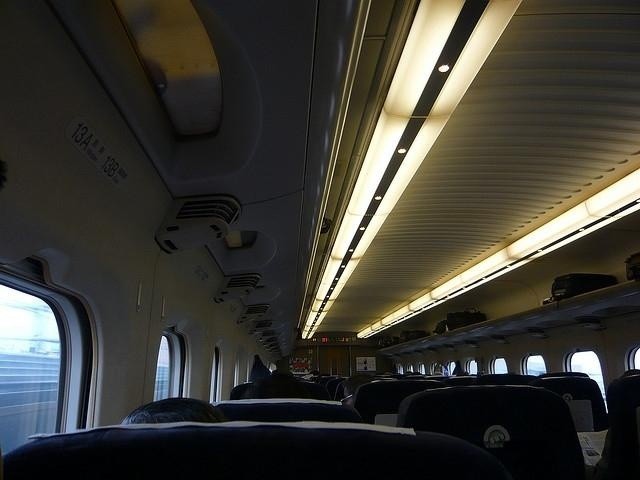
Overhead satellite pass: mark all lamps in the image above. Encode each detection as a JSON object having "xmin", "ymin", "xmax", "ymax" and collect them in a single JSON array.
[
  {"xmin": 297, "ymin": 0, "xmax": 523, "ymax": 342},
  {"xmin": 357, "ymin": 169, "xmax": 640, "ymax": 340}
]
